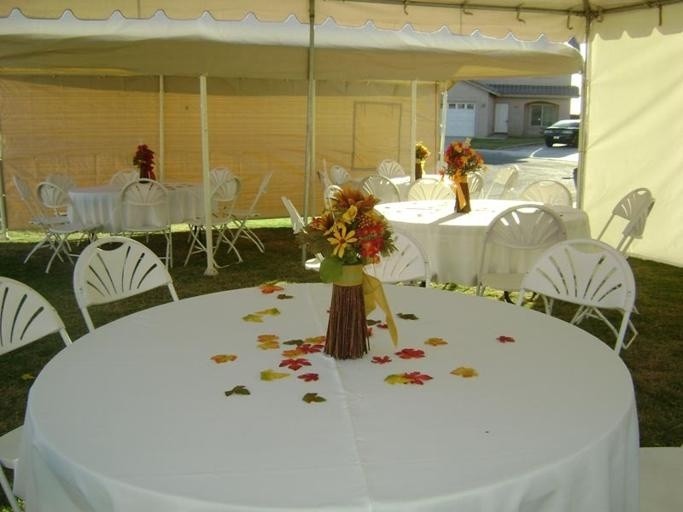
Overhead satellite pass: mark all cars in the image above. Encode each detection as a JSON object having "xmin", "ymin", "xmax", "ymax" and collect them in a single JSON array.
[{"xmin": 543, "ymin": 118, "xmax": 579, "ymax": 148}]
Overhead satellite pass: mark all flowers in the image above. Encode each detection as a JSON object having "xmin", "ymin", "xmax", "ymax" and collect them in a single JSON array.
[
  {"xmin": 133, "ymin": 144, "xmax": 154, "ymax": 164},
  {"xmin": 294, "ymin": 188, "xmax": 397, "ymax": 283},
  {"xmin": 415, "ymin": 143, "xmax": 430, "ymax": 162},
  {"xmin": 444, "ymin": 141, "xmax": 489, "ymax": 176}
]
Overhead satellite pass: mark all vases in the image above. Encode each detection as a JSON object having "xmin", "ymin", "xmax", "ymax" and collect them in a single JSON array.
[
  {"xmin": 139, "ymin": 165, "xmax": 149, "ymax": 183},
  {"xmin": 322, "ymin": 264, "xmax": 371, "ymax": 361}
]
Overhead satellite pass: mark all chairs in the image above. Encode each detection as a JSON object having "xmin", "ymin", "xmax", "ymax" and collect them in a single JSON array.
[
  {"xmin": 11, "ymin": 168, "xmax": 273, "ymax": 273},
  {"xmin": 1, "ymin": 277, "xmax": 72, "ymax": 512},
  {"xmin": 72, "ymin": 236, "xmax": 180, "ymax": 331}
]
